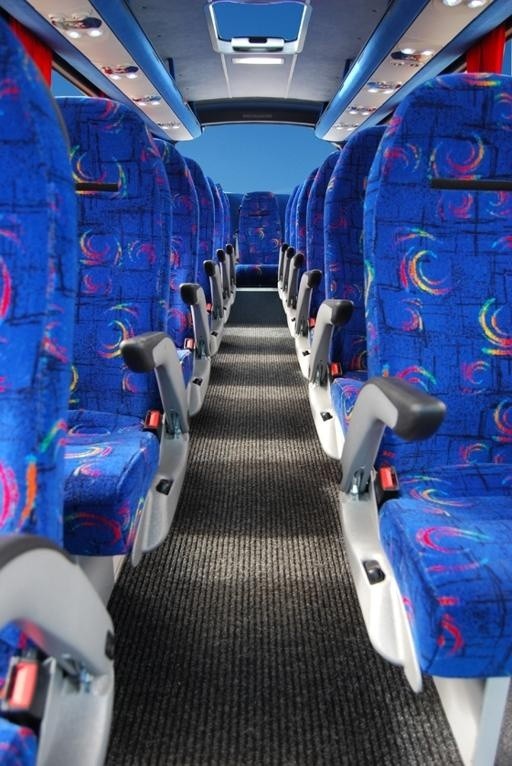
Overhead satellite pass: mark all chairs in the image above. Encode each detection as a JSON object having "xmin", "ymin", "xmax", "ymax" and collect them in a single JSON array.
[
  {"xmin": 234, "ymin": 192, "xmax": 281, "ymax": 285},
  {"xmin": 0, "ymin": 24, "xmax": 116, "ymax": 761},
  {"xmin": 336, "ymin": 71, "xmax": 512, "ymax": 766},
  {"xmin": 279, "ymin": 125, "xmax": 384, "ymax": 460},
  {"xmin": 153, "ymin": 140, "xmax": 233, "ymax": 419},
  {"xmin": 55, "ymin": 96, "xmax": 188, "ymax": 601}
]
